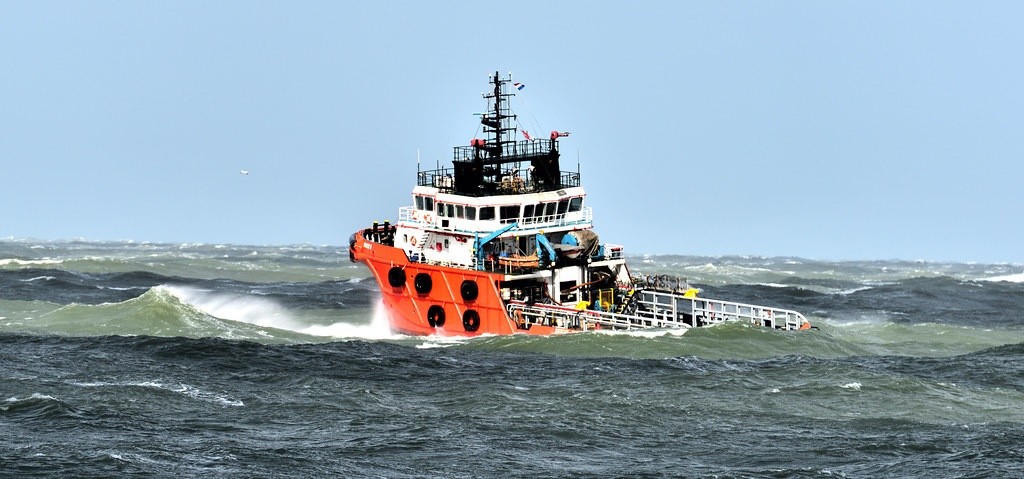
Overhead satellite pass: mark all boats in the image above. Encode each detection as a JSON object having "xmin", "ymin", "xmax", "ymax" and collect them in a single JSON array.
[{"xmin": 349, "ymin": 68, "xmax": 809, "ymax": 348}]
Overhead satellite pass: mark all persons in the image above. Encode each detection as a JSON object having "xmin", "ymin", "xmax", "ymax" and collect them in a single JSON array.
[{"xmin": 585, "ymin": 295, "xmax": 603, "ymax": 330}]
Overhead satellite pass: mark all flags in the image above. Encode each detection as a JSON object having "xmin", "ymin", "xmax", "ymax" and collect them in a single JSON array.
[{"xmin": 513, "ymin": 82, "xmax": 525, "ymax": 91}]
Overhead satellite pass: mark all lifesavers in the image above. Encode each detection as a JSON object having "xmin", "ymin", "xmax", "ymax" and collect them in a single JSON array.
[
  {"xmin": 410, "ymin": 236, "xmax": 417, "ymax": 246},
  {"xmin": 424, "ymin": 214, "xmax": 432, "ymax": 223}
]
